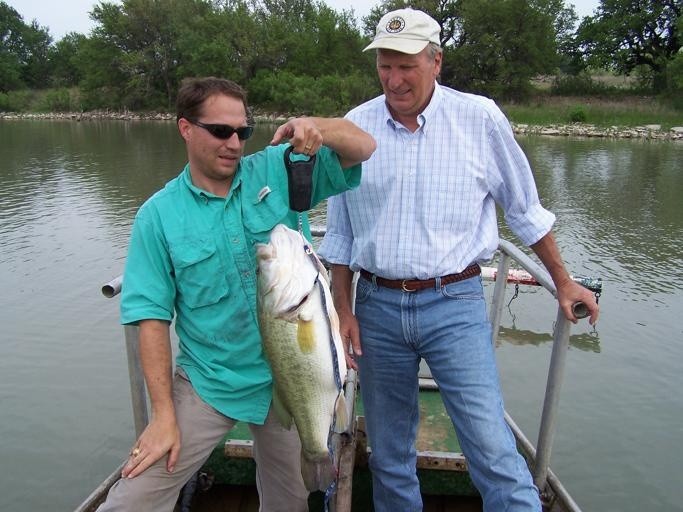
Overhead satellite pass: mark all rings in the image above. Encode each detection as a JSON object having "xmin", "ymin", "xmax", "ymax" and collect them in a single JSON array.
[
  {"xmin": 305, "ymin": 145, "xmax": 313, "ymax": 150},
  {"xmin": 133, "ymin": 448, "xmax": 141, "ymax": 456}
]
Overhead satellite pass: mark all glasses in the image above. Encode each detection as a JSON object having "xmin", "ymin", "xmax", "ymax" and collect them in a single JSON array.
[{"xmin": 195, "ymin": 117, "xmax": 253, "ymax": 140}]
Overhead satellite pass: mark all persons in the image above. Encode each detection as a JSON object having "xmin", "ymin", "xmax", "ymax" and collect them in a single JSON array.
[
  {"xmin": 318, "ymin": 8, "xmax": 599, "ymax": 512},
  {"xmin": 96, "ymin": 76, "xmax": 378, "ymax": 512}
]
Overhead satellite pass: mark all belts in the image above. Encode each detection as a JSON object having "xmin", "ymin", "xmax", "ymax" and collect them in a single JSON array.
[{"xmin": 359, "ymin": 262, "xmax": 482, "ymax": 292}]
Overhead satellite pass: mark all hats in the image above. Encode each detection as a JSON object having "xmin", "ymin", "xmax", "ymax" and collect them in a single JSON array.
[{"xmin": 362, "ymin": 7, "xmax": 442, "ymax": 56}]
